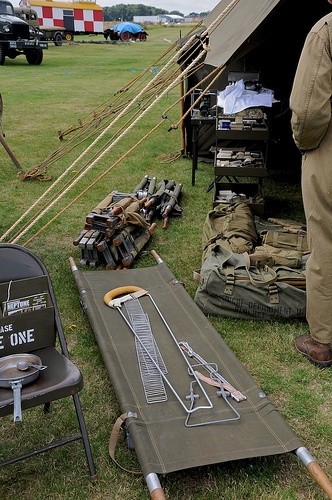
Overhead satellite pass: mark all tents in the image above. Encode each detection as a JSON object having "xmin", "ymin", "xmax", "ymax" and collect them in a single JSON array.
[{"xmin": 177, "ymin": 1, "xmax": 332, "ymax": 159}]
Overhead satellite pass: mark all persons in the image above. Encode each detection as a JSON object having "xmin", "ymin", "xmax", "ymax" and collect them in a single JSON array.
[{"xmin": 289, "ymin": 0, "xmax": 332, "ymax": 367}]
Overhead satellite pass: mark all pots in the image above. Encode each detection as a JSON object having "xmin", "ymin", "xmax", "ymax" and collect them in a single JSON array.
[{"xmin": 0, "ymin": 353, "xmax": 42, "ymax": 423}]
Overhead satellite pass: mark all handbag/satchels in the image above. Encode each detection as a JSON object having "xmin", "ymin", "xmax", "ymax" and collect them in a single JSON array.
[{"xmin": 194, "ymin": 202, "xmax": 307, "ymax": 320}]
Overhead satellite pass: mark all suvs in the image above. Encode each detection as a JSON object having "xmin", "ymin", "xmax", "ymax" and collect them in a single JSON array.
[{"xmin": 0, "ymin": 0, "xmax": 67, "ymax": 66}]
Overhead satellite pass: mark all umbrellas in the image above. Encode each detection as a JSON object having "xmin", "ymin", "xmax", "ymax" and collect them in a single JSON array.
[{"xmin": 110, "ymin": 22, "xmax": 145, "ymax": 44}]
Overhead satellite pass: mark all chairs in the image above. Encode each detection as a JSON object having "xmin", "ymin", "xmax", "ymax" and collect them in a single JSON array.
[{"xmin": 0, "ymin": 242, "xmax": 98, "ymax": 486}]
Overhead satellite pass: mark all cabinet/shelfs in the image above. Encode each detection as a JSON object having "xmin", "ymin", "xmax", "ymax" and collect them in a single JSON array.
[{"xmin": 214, "ymin": 106, "xmax": 271, "ymax": 218}]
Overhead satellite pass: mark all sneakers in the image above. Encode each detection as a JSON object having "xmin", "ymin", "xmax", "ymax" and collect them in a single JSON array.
[{"xmin": 295, "ymin": 333, "xmax": 332, "ymax": 367}]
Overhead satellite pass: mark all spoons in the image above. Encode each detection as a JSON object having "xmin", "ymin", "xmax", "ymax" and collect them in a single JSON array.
[{"xmin": 17, "ymin": 361, "xmax": 48, "ymax": 371}]
